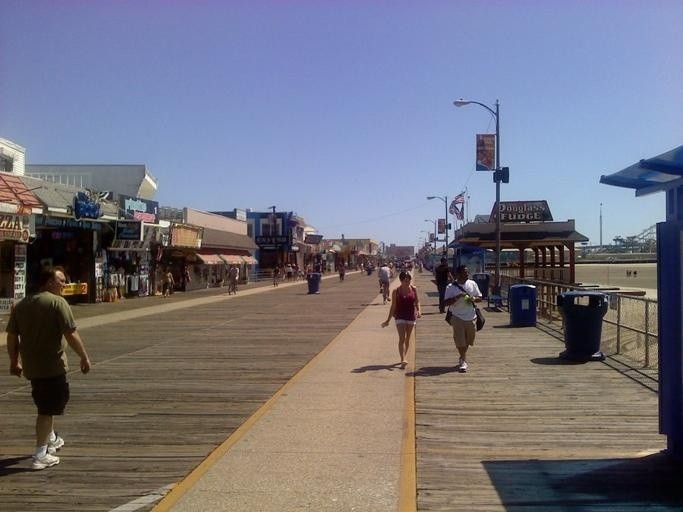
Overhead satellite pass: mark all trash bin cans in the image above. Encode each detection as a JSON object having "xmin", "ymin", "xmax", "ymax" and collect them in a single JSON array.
[
  {"xmin": 472, "ymin": 273, "xmax": 490, "ymax": 300},
  {"xmin": 557, "ymin": 291, "xmax": 608, "ymax": 361},
  {"xmin": 306, "ymin": 273, "xmax": 321, "ymax": 294},
  {"xmin": 509, "ymin": 284, "xmax": 537, "ymax": 326}
]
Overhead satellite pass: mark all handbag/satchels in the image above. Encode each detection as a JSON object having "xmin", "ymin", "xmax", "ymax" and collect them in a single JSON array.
[{"xmin": 451, "ymin": 281, "xmax": 486, "ymax": 333}]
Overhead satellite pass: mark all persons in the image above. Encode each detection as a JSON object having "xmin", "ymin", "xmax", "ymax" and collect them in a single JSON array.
[
  {"xmin": 227, "ymin": 264, "xmax": 239, "ymax": 291},
  {"xmin": 5, "ymin": 265, "xmax": 91, "ymax": 471},
  {"xmin": 160, "ymin": 268, "xmax": 175, "ymax": 296},
  {"xmin": 274, "ymin": 254, "xmax": 483, "ymax": 373}
]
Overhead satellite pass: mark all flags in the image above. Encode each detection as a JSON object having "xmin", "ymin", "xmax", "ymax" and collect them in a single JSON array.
[{"xmin": 449, "ymin": 193, "xmax": 465, "ymax": 220}]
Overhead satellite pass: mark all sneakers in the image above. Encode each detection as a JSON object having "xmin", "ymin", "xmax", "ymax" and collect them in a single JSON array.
[
  {"xmin": 29, "ymin": 452, "xmax": 61, "ymax": 470},
  {"xmin": 45, "ymin": 432, "xmax": 67, "ymax": 451},
  {"xmin": 459, "ymin": 356, "xmax": 470, "ymax": 371}
]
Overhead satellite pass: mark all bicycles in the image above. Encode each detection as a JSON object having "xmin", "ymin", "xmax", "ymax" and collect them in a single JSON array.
[
  {"xmin": 379, "ymin": 281, "xmax": 389, "ymax": 305},
  {"xmin": 273, "ymin": 275, "xmax": 279, "ymax": 287},
  {"xmin": 226, "ymin": 277, "xmax": 239, "ymax": 295}
]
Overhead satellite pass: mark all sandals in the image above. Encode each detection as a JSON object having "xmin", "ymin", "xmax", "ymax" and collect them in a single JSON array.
[{"xmin": 399, "ymin": 360, "xmax": 410, "ymax": 370}]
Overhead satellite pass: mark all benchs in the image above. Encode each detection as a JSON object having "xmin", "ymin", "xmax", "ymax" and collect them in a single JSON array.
[{"xmin": 487, "ymin": 294, "xmax": 510, "ymax": 313}]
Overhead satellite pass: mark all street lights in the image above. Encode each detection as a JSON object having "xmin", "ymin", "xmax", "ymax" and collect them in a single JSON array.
[
  {"xmin": 453, "ymin": 95, "xmax": 505, "ymax": 313},
  {"xmin": 267, "ymin": 206, "xmax": 279, "ymax": 267},
  {"xmin": 598, "ymin": 203, "xmax": 604, "ymax": 249},
  {"xmin": 424, "ymin": 218, "xmax": 438, "ymax": 257},
  {"xmin": 418, "ymin": 228, "xmax": 431, "ymax": 253},
  {"xmin": 427, "ymin": 194, "xmax": 449, "ymax": 266}
]
